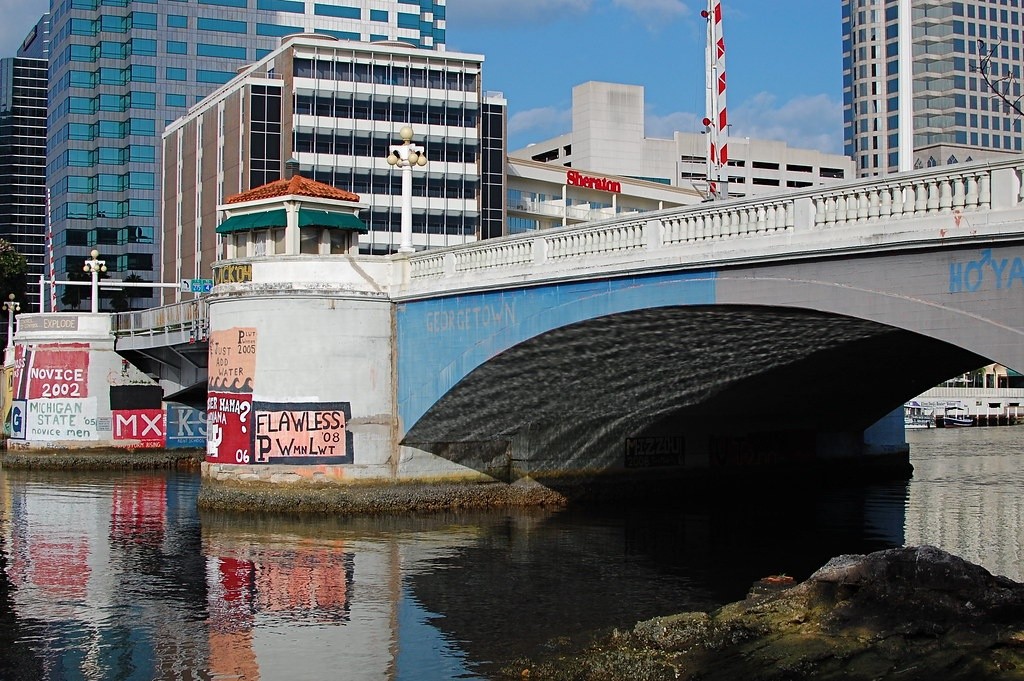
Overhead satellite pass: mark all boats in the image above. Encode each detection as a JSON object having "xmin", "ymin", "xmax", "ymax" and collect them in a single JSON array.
[
  {"xmin": 943, "ymin": 406, "xmax": 974, "ymax": 427},
  {"xmin": 904, "ymin": 401, "xmax": 937, "ymax": 429}
]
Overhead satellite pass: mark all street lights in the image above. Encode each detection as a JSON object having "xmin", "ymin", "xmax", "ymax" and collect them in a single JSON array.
[
  {"xmin": 2, "ymin": 293, "xmax": 20, "ymax": 366},
  {"xmin": 388, "ymin": 126, "xmax": 426, "ymax": 250},
  {"xmin": 83, "ymin": 250, "xmax": 107, "ymax": 311}
]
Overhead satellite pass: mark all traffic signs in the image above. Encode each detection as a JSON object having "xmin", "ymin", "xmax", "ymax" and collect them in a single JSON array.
[{"xmin": 180, "ymin": 279, "xmax": 190, "ymax": 292}]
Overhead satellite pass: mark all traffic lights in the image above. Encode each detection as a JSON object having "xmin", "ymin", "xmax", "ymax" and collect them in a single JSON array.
[
  {"xmin": 199, "ymin": 318, "xmax": 206, "ymax": 329},
  {"xmin": 192, "ymin": 319, "xmax": 200, "ymax": 330}
]
